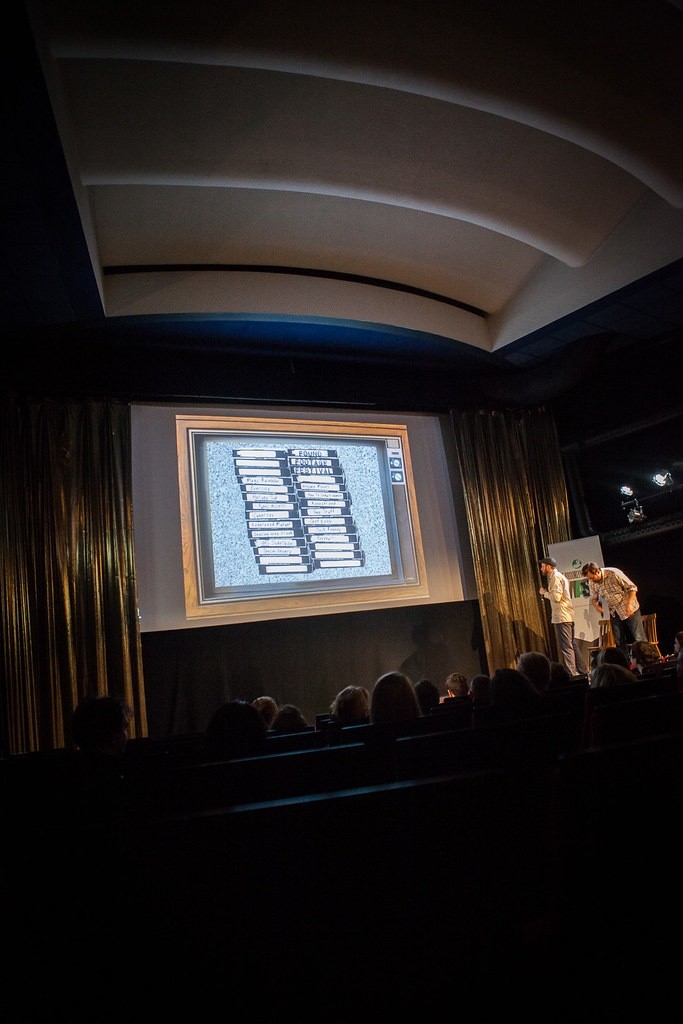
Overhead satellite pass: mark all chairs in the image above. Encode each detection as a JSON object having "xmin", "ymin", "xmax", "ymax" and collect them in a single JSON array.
[
  {"xmin": 588, "ymin": 619, "xmax": 617, "ymax": 673},
  {"xmin": 626, "ymin": 613, "xmax": 664, "ymax": 662}
]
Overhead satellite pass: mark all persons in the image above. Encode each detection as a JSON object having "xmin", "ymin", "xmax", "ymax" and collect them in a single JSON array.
[
  {"xmin": 467, "ymin": 674, "xmax": 490, "ymax": 708},
  {"xmin": 581, "ymin": 563, "xmax": 649, "ymax": 658},
  {"xmin": 413, "ymin": 680, "xmax": 440, "ymax": 710},
  {"xmin": 443, "ymin": 673, "xmax": 469, "ymax": 697},
  {"xmin": 674, "ymin": 630, "xmax": 683, "ymax": 662},
  {"xmin": 517, "ymin": 651, "xmax": 551, "ymax": 692},
  {"xmin": 630, "ymin": 640, "xmax": 660, "ymax": 675},
  {"xmin": 271, "ymin": 705, "xmax": 307, "ymax": 732},
  {"xmin": 549, "ymin": 662, "xmax": 570, "ymax": 683},
  {"xmin": 73, "ymin": 696, "xmax": 130, "ymax": 748},
  {"xmin": 250, "ymin": 696, "xmax": 277, "ymax": 731},
  {"xmin": 587, "ymin": 648, "xmax": 637, "ymax": 688},
  {"xmin": 328, "ymin": 685, "xmax": 370, "ymax": 722},
  {"xmin": 538, "ymin": 557, "xmax": 580, "ymax": 675},
  {"xmin": 204, "ymin": 700, "xmax": 268, "ymax": 759},
  {"xmin": 489, "ymin": 668, "xmax": 539, "ymax": 706},
  {"xmin": 370, "ymin": 672, "xmax": 422, "ymax": 726}
]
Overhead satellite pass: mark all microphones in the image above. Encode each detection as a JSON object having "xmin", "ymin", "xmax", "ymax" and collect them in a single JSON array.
[
  {"xmin": 540, "ymin": 585, "xmax": 546, "ymax": 602},
  {"xmin": 597, "ymin": 601, "xmax": 604, "ymax": 619}
]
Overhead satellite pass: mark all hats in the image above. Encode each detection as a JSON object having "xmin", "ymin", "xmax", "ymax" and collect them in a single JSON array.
[{"xmin": 538, "ymin": 557, "xmax": 556, "ymax": 566}]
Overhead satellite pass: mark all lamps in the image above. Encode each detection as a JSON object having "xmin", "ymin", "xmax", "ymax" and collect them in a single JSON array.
[
  {"xmin": 653, "ymin": 469, "xmax": 677, "ymax": 486},
  {"xmin": 627, "ymin": 509, "xmax": 648, "ymax": 524},
  {"xmin": 620, "ymin": 476, "xmax": 638, "ymax": 496}
]
